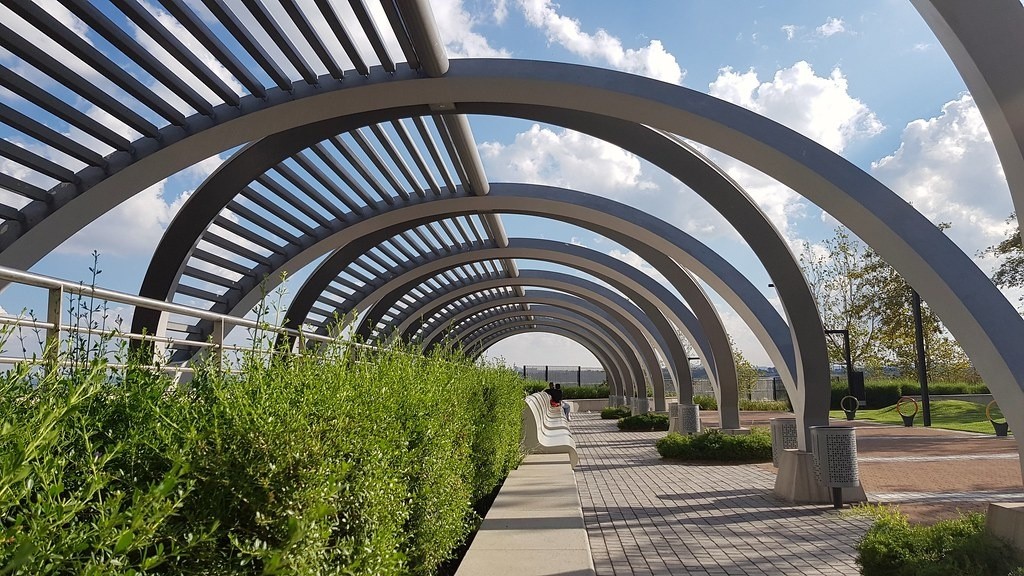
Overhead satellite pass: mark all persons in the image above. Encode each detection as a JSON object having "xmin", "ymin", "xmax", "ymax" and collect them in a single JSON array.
[{"xmin": 544, "ymin": 382, "xmax": 573, "ymax": 422}]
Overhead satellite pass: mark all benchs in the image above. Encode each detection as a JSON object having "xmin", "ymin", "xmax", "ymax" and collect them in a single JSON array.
[{"xmin": 524, "ymin": 391, "xmax": 579, "ymax": 469}]
